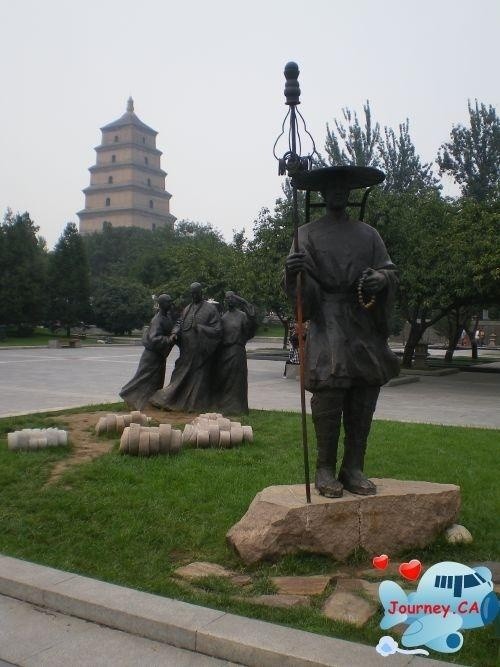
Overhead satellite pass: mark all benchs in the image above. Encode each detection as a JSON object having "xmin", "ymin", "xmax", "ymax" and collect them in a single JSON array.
[{"xmin": 47, "ymin": 338, "xmax": 82, "ymax": 348}]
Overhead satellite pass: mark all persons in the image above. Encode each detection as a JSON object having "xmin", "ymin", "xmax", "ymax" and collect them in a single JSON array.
[
  {"xmin": 213, "ymin": 291, "xmax": 257, "ymax": 416},
  {"xmin": 283, "ymin": 323, "xmax": 300, "ymax": 377},
  {"xmin": 119, "ymin": 294, "xmax": 174, "ymax": 411},
  {"xmin": 281, "ymin": 166, "xmax": 400, "ymax": 495},
  {"xmin": 461, "ymin": 327, "xmax": 486, "ymax": 347},
  {"xmin": 150, "ymin": 282, "xmax": 224, "ymax": 412}
]
